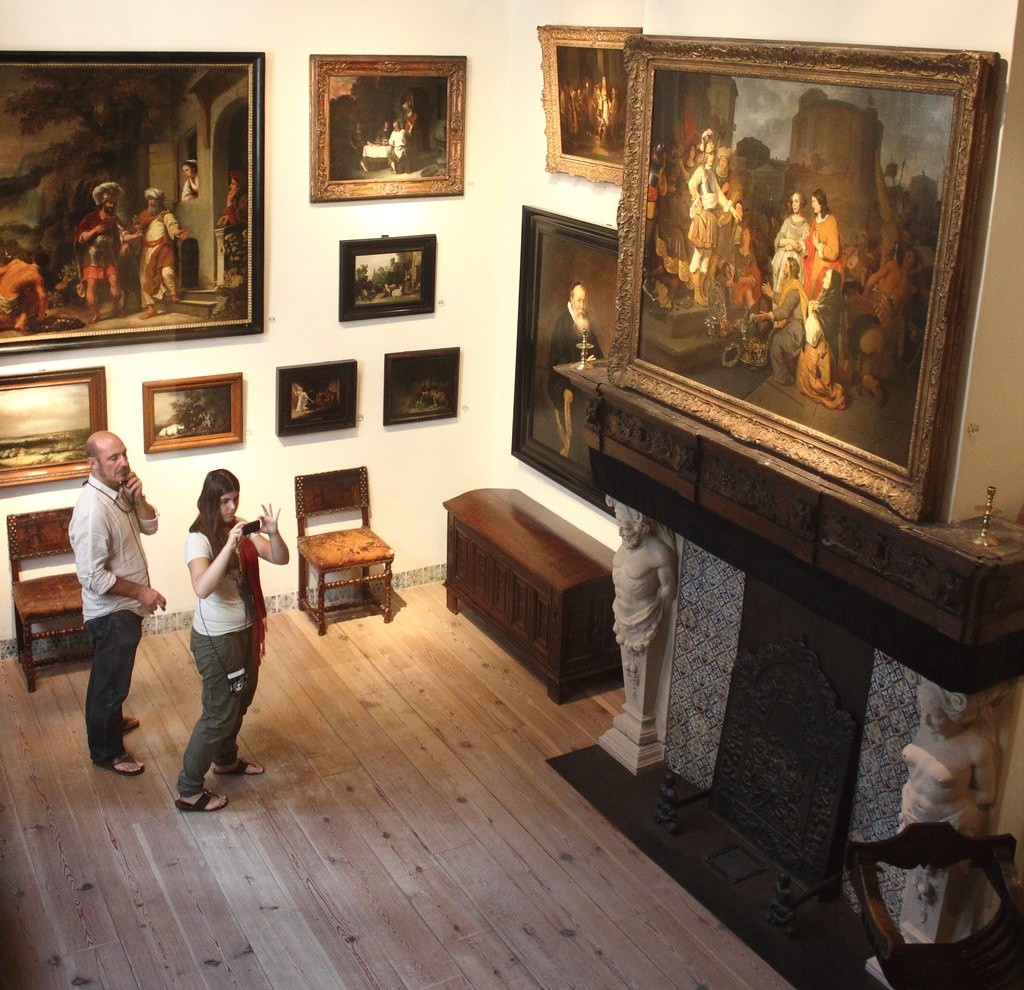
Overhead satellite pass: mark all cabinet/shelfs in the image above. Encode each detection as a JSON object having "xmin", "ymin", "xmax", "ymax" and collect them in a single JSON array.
[{"xmin": 442, "ymin": 488, "xmax": 624, "ymax": 706}]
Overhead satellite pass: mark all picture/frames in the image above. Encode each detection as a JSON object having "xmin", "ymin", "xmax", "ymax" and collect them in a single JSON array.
[
  {"xmin": 383, "ymin": 347, "xmax": 460, "ymax": 426},
  {"xmin": 510, "ymin": 204, "xmax": 617, "ymax": 520},
  {"xmin": 142, "ymin": 372, "xmax": 243, "ymax": 454},
  {"xmin": 0, "ymin": 365, "xmax": 109, "ymax": 487},
  {"xmin": 607, "ymin": 33, "xmax": 1000, "ymax": 526},
  {"xmin": 537, "ymin": 24, "xmax": 644, "ymax": 186},
  {"xmin": 0, "ymin": 51, "xmax": 266, "ymax": 357},
  {"xmin": 275, "ymin": 358, "xmax": 357, "ymax": 437},
  {"xmin": 309, "ymin": 53, "xmax": 468, "ymax": 204},
  {"xmin": 338, "ymin": 233, "xmax": 437, "ymax": 324}
]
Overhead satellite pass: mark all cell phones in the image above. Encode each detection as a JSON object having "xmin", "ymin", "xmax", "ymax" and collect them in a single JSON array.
[{"xmin": 242, "ymin": 521, "xmax": 260, "ymax": 535}]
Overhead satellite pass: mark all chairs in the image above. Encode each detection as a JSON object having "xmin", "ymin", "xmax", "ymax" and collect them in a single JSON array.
[
  {"xmin": 6, "ymin": 507, "xmax": 95, "ymax": 694},
  {"xmin": 294, "ymin": 465, "xmax": 395, "ymax": 637}
]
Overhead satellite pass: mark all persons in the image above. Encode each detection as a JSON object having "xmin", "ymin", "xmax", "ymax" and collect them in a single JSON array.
[
  {"xmin": 612, "ymin": 504, "xmax": 676, "ymax": 652},
  {"xmin": 902, "ymin": 682, "xmax": 996, "ymax": 829},
  {"xmin": 176, "ymin": 469, "xmax": 289, "ymax": 810},
  {"xmin": 68, "ymin": 431, "xmax": 166, "ymax": 776}
]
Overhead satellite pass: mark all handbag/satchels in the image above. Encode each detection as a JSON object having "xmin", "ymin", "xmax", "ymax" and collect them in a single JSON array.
[{"xmin": 225, "ymin": 668, "xmax": 250, "ymax": 697}]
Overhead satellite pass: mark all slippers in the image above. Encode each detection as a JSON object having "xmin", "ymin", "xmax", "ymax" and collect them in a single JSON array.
[
  {"xmin": 121, "ymin": 716, "xmax": 140, "ymax": 730},
  {"xmin": 213, "ymin": 758, "xmax": 265, "ymax": 776},
  {"xmin": 92, "ymin": 753, "xmax": 145, "ymax": 776},
  {"xmin": 175, "ymin": 788, "xmax": 228, "ymax": 811}
]
